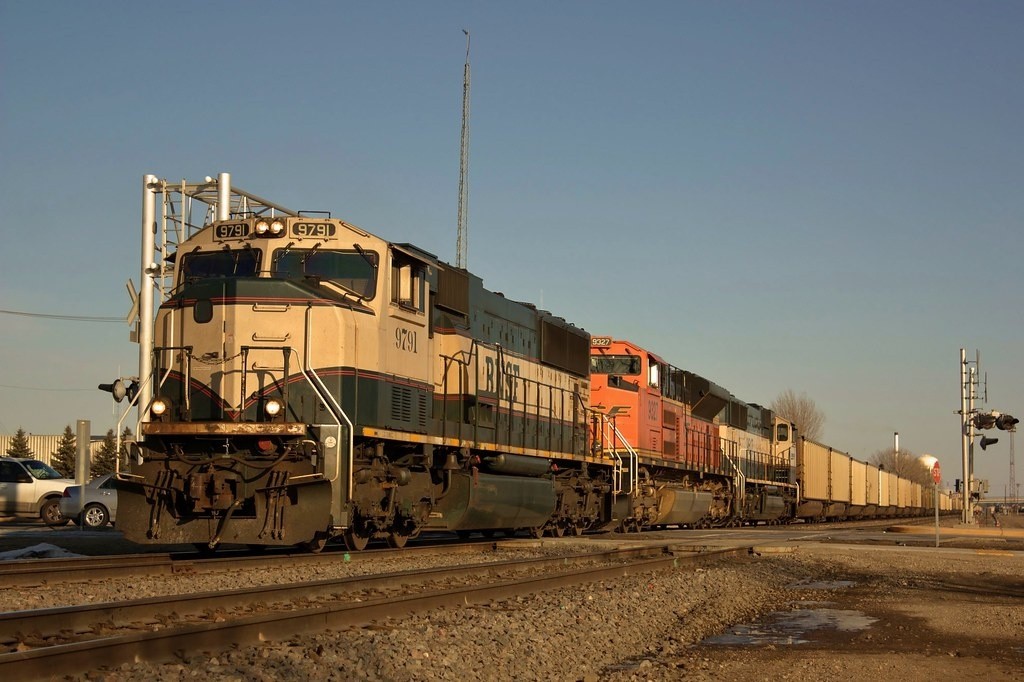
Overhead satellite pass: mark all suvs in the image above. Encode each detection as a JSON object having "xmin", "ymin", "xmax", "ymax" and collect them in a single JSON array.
[{"xmin": 0, "ymin": 455, "xmax": 75, "ymax": 527}]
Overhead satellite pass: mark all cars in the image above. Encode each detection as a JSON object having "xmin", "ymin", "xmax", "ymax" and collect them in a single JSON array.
[{"xmin": 59, "ymin": 470, "xmax": 129, "ymax": 531}]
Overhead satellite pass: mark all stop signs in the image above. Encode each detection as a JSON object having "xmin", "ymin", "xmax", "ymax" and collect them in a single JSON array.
[{"xmin": 932, "ymin": 462, "xmax": 941, "ymax": 484}]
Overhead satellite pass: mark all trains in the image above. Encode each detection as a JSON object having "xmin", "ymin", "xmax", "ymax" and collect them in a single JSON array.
[{"xmin": 99, "ymin": 173, "xmax": 963, "ymax": 559}]
